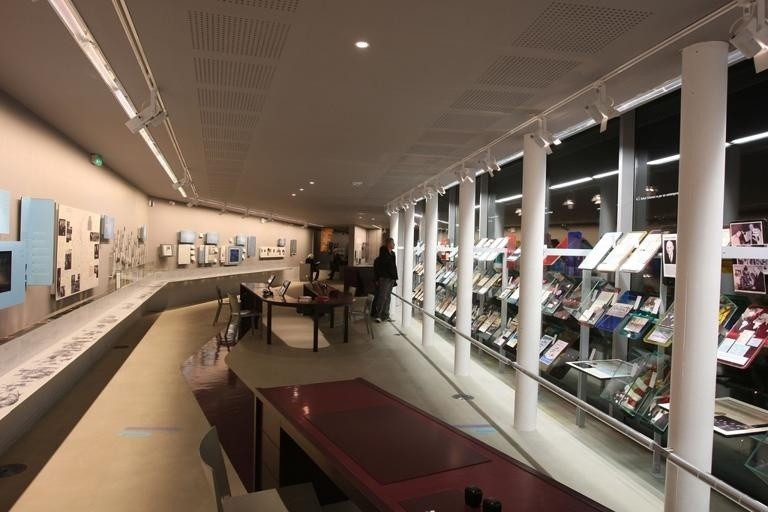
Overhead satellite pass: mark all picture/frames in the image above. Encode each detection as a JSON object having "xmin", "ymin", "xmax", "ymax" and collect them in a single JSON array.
[
  {"xmin": 565, "ymin": 359, "xmax": 636, "ymax": 380},
  {"xmin": 268, "ymin": 273, "xmax": 276, "ymax": 288},
  {"xmin": 278, "ymin": 280, "xmax": 291, "ymax": 297},
  {"xmin": 657, "ymin": 397, "xmax": 768, "ymax": 437}
]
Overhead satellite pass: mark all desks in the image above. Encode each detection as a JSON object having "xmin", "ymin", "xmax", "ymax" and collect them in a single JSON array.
[
  {"xmin": 255, "ymin": 377, "xmax": 614, "ymax": 512},
  {"xmin": 240, "ymin": 282, "xmax": 356, "ymax": 353}
]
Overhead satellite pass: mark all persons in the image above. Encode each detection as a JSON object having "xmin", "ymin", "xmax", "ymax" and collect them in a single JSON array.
[
  {"xmin": 664, "ymin": 240, "xmax": 675, "ymax": 264},
  {"xmin": 731, "ymin": 230, "xmax": 741, "ymax": 244},
  {"xmin": 740, "ymin": 266, "xmax": 755, "ymax": 289},
  {"xmin": 370, "ymin": 245, "xmax": 387, "ymax": 318},
  {"xmin": 741, "ymin": 224, "xmax": 761, "ymax": 246},
  {"xmin": 375, "ymin": 238, "xmax": 398, "ymax": 322},
  {"xmin": 749, "ymin": 266, "xmax": 764, "ymax": 291}
]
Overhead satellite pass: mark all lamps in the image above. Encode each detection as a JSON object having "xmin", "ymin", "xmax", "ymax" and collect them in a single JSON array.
[
  {"xmin": 479, "ymin": 143, "xmax": 501, "ymax": 178},
  {"xmin": 728, "ymin": 0, "xmax": 768, "ymax": 73},
  {"xmin": 187, "ymin": 195, "xmax": 199, "ymax": 208},
  {"xmin": 91, "ymin": 153, "xmax": 103, "ymax": 166},
  {"xmin": 384, "ymin": 175, "xmax": 446, "ymax": 217},
  {"xmin": 584, "ymin": 78, "xmax": 619, "ymax": 132},
  {"xmin": 50, "ymin": 0, "xmax": 188, "ymax": 197},
  {"xmin": 454, "ymin": 158, "xmax": 474, "ymax": 184},
  {"xmin": 125, "ymin": 89, "xmax": 167, "ymax": 134},
  {"xmin": 172, "ymin": 170, "xmax": 192, "ymax": 190},
  {"xmin": 531, "ymin": 112, "xmax": 562, "ymax": 155}
]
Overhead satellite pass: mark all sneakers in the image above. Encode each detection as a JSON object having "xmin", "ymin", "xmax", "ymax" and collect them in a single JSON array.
[
  {"xmin": 382, "ymin": 316, "xmax": 396, "ymax": 322},
  {"xmin": 375, "ymin": 317, "xmax": 381, "ymax": 323}
]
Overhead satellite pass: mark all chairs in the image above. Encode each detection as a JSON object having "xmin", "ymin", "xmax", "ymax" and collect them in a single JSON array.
[
  {"xmin": 225, "ymin": 295, "xmax": 263, "ymax": 336},
  {"xmin": 199, "ymin": 425, "xmax": 322, "ymax": 512},
  {"xmin": 348, "ymin": 293, "xmax": 374, "ymax": 340},
  {"xmin": 213, "ymin": 286, "xmax": 242, "ymax": 326}
]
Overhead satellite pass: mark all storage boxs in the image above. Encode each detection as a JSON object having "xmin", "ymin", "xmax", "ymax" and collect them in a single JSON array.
[
  {"xmin": 599, "ymin": 346, "xmax": 672, "ymax": 433},
  {"xmin": 542, "ymin": 270, "xmax": 574, "ymax": 316},
  {"xmin": 564, "ymin": 278, "xmax": 614, "ymax": 325},
  {"xmin": 745, "ymin": 432, "xmax": 768, "ymax": 487}
]
